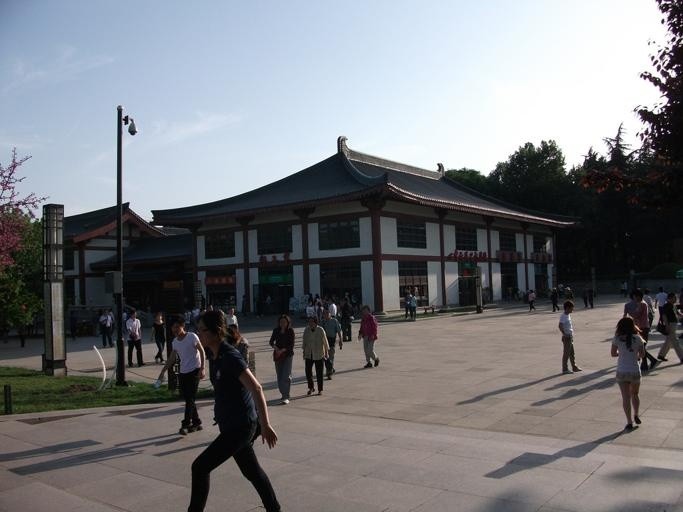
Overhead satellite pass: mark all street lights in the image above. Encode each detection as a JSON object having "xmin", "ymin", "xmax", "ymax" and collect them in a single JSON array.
[
  {"xmin": 113, "ymin": 104, "xmax": 140, "ymax": 385},
  {"xmin": 40, "ymin": 204, "xmax": 68, "ymax": 377}
]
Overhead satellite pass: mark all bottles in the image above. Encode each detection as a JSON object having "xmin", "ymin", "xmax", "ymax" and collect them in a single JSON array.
[{"xmin": 152, "ymin": 375, "xmax": 165, "ymax": 389}]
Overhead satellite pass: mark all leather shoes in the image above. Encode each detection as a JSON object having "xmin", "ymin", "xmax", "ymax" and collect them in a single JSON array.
[
  {"xmin": 641, "ymin": 366, "xmax": 648, "ymax": 371},
  {"xmin": 650, "ymin": 359, "xmax": 657, "ymax": 369},
  {"xmin": 658, "ymin": 355, "xmax": 667, "ymax": 361}
]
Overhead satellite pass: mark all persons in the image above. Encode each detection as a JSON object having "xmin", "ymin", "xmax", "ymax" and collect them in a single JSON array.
[
  {"xmin": 509, "ymin": 280, "xmax": 682, "ymax": 374},
  {"xmin": 611, "ymin": 318, "xmax": 646, "ymax": 430},
  {"xmin": 358, "ymin": 306, "xmax": 380, "ymax": 367},
  {"xmin": 405, "ymin": 293, "xmax": 416, "ymax": 321},
  {"xmin": 100, "ymin": 292, "xmax": 357, "ymax": 435},
  {"xmin": 187, "ymin": 309, "xmax": 281, "ymax": 512}
]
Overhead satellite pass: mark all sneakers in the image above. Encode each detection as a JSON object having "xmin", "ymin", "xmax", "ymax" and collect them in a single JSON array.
[
  {"xmin": 365, "ymin": 363, "xmax": 372, "ymax": 368},
  {"xmin": 573, "ymin": 367, "xmax": 581, "ymax": 372},
  {"xmin": 374, "ymin": 359, "xmax": 379, "ymax": 366},
  {"xmin": 189, "ymin": 425, "xmax": 202, "ymax": 431},
  {"xmin": 283, "ymin": 399, "xmax": 289, "ymax": 404},
  {"xmin": 180, "ymin": 425, "xmax": 189, "ymax": 435},
  {"xmin": 280, "ymin": 398, "xmax": 283, "ymax": 405},
  {"xmin": 318, "ymin": 391, "xmax": 322, "ymax": 395},
  {"xmin": 563, "ymin": 370, "xmax": 573, "ymax": 374},
  {"xmin": 308, "ymin": 389, "xmax": 315, "ymax": 395}
]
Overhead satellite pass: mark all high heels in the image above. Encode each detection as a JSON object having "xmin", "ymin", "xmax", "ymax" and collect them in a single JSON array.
[
  {"xmin": 625, "ymin": 424, "xmax": 633, "ymax": 431},
  {"xmin": 635, "ymin": 416, "xmax": 641, "ymax": 424}
]
